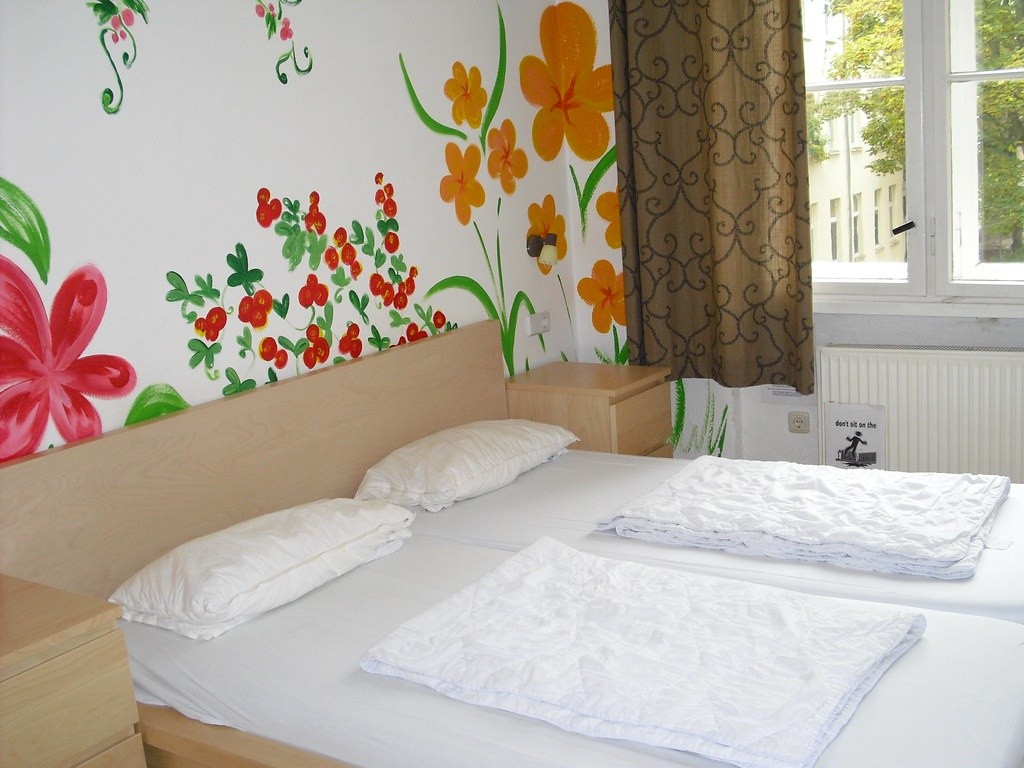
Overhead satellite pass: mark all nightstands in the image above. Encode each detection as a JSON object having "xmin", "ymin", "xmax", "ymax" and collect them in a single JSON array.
[
  {"xmin": 506, "ymin": 361, "xmax": 674, "ymax": 458},
  {"xmin": 0, "ymin": 573, "xmax": 147, "ymax": 768}
]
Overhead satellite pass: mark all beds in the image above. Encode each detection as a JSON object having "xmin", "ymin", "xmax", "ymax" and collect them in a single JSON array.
[{"xmin": 0, "ymin": 319, "xmax": 1024, "ymax": 768}]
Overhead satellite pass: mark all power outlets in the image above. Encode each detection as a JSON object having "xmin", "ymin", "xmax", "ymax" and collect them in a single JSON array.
[{"xmin": 788, "ymin": 411, "xmax": 810, "ymax": 434}]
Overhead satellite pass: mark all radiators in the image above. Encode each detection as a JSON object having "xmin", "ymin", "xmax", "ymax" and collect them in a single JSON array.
[{"xmin": 819, "ymin": 341, "xmax": 1024, "ymax": 483}]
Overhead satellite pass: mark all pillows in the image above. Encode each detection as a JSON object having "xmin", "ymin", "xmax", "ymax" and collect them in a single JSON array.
[
  {"xmin": 108, "ymin": 496, "xmax": 418, "ymax": 641},
  {"xmin": 354, "ymin": 416, "xmax": 580, "ymax": 514}
]
